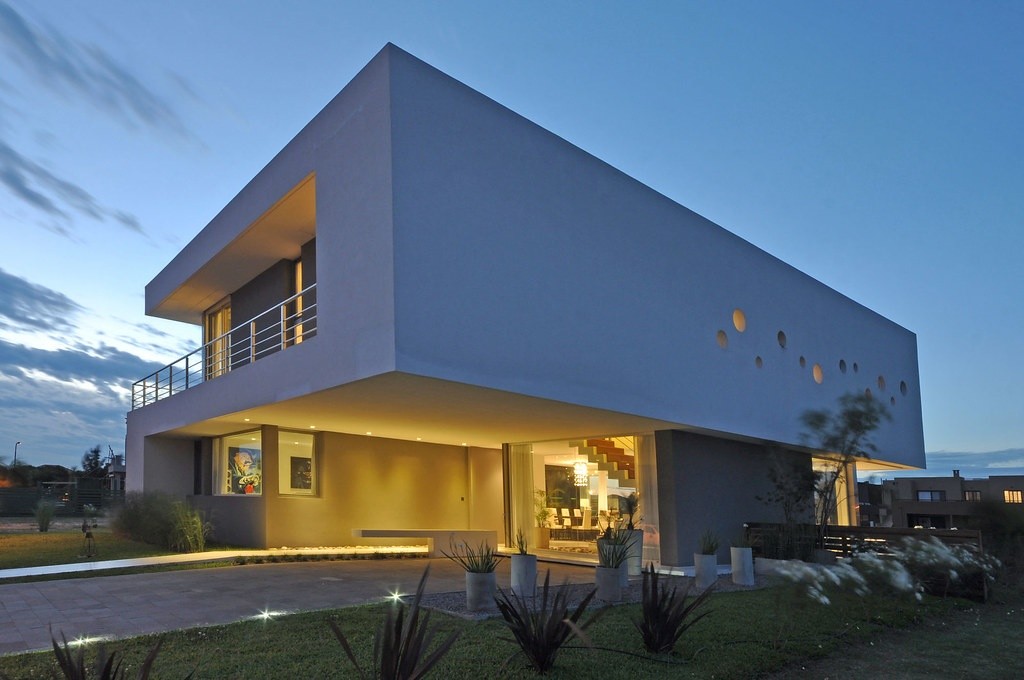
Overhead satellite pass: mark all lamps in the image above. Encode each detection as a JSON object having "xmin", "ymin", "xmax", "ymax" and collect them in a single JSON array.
[{"xmin": 572, "ymin": 462, "xmax": 588, "ymax": 487}]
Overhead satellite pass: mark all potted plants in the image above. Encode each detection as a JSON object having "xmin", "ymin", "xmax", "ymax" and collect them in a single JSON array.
[
  {"xmin": 594, "ymin": 532, "xmax": 640, "ymax": 600},
  {"xmin": 620, "ymin": 493, "xmax": 644, "ymax": 576},
  {"xmin": 440, "ymin": 532, "xmax": 504, "ymax": 610},
  {"xmin": 726, "ymin": 533, "xmax": 757, "ymax": 586},
  {"xmin": 533, "ymin": 487, "xmax": 568, "ymax": 548},
  {"xmin": 597, "ymin": 511, "xmax": 629, "ymax": 589},
  {"xmin": 692, "ymin": 529, "xmax": 720, "ymax": 589},
  {"xmin": 508, "ymin": 529, "xmax": 537, "ymax": 598}
]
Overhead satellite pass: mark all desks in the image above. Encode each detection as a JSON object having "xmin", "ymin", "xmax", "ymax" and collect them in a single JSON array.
[{"xmin": 554, "ymin": 515, "xmax": 599, "ymax": 541}]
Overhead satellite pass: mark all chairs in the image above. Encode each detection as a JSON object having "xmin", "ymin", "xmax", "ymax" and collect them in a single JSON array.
[
  {"xmin": 561, "ymin": 508, "xmax": 575, "ymax": 538},
  {"xmin": 573, "ymin": 508, "xmax": 583, "ymax": 538},
  {"xmin": 545, "ymin": 508, "xmax": 560, "ymax": 539},
  {"xmin": 545, "ymin": 514, "xmax": 567, "ymax": 540},
  {"xmin": 572, "ymin": 509, "xmax": 593, "ymax": 540}
]
